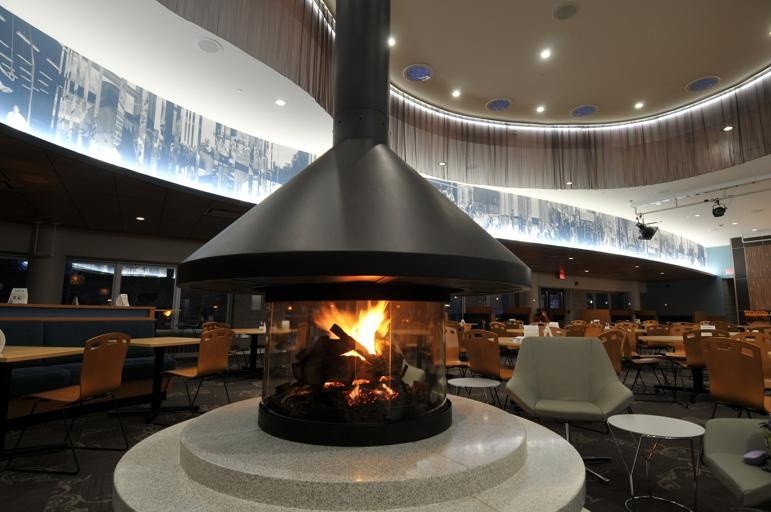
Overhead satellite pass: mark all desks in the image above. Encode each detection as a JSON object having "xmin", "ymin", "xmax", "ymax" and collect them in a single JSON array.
[
  {"xmin": 0, "ymin": 345, "xmax": 84, "ymax": 460},
  {"xmin": 393, "ymin": 329, "xmax": 431, "ymax": 368},
  {"xmin": 607, "ymin": 414, "xmax": 706, "ymax": 512},
  {"xmin": 107, "ymin": 336, "xmax": 210, "ymax": 424}
]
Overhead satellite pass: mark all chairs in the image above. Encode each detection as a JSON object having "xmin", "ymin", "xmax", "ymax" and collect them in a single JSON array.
[
  {"xmin": 5, "ymin": 333, "xmax": 131, "ymax": 474},
  {"xmin": 202, "ymin": 322, "xmax": 307, "ymax": 377},
  {"xmin": 446, "ymin": 320, "xmax": 771, "ymax": 506},
  {"xmin": 149, "ymin": 328, "xmax": 234, "ymax": 427}
]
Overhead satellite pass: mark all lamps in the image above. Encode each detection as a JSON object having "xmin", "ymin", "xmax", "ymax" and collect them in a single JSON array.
[
  {"xmin": 712, "ymin": 198, "xmax": 727, "ymax": 217},
  {"xmin": 636, "ymin": 213, "xmax": 658, "ymax": 240}
]
